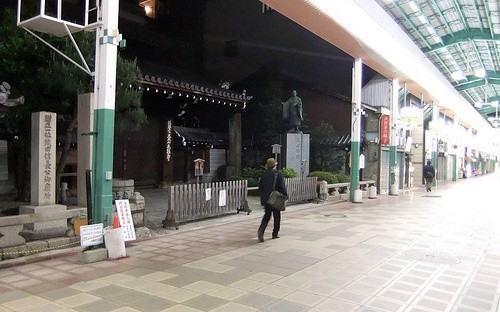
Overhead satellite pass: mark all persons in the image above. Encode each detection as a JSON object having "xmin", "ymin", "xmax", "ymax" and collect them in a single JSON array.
[
  {"xmin": 255, "ymin": 158, "xmax": 288, "ymax": 243},
  {"xmin": 280, "ymin": 88, "xmax": 304, "ymax": 133},
  {"xmin": 422, "ymin": 160, "xmax": 435, "ymax": 192}
]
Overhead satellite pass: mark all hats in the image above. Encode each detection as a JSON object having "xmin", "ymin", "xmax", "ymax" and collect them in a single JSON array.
[{"xmin": 264, "ymin": 158, "xmax": 278, "ymax": 169}]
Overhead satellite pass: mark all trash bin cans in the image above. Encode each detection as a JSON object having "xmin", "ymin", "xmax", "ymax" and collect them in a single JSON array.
[{"xmin": 112, "ymin": 178, "xmax": 134, "ymax": 205}]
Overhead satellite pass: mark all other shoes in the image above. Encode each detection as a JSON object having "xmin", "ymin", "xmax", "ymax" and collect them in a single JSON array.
[
  {"xmin": 258, "ymin": 228, "xmax": 264, "ymax": 241},
  {"xmin": 272, "ymin": 234, "xmax": 280, "ymax": 239}
]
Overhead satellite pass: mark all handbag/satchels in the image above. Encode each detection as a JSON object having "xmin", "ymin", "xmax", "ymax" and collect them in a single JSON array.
[
  {"xmin": 267, "ymin": 171, "xmax": 288, "ymax": 211},
  {"xmin": 425, "ymin": 172, "xmax": 434, "ymax": 178}
]
